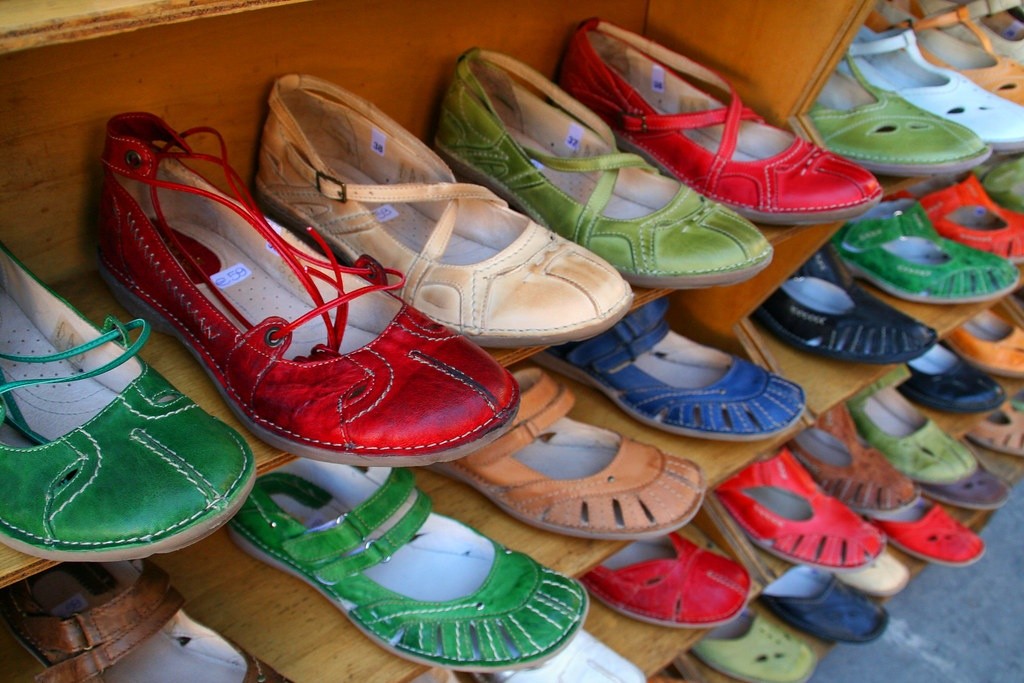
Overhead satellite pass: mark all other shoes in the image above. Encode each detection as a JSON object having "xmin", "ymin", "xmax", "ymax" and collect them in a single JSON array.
[
  {"xmin": 227, "ymin": 456, "xmax": 589, "ymax": 673},
  {"xmin": 427, "ymin": 367, "xmax": 707, "ymax": 541},
  {"xmin": 556, "ymin": 16, "xmax": 884, "ymax": 226},
  {"xmin": 691, "ymin": 2, "xmax": 1022, "ymax": 683},
  {"xmin": 1, "ymin": 244, "xmax": 257, "ymax": 561},
  {"xmin": 256, "ymin": 72, "xmax": 634, "ymax": 349},
  {"xmin": 435, "ymin": 48, "xmax": 773, "ymax": 289},
  {"xmin": 581, "ymin": 531, "xmax": 752, "ymax": 628},
  {"xmin": 473, "ymin": 628, "xmax": 646, "ymax": 683},
  {"xmin": 93, "ymin": 112, "xmax": 520, "ymax": 468},
  {"xmin": 0, "ymin": 561, "xmax": 294, "ymax": 683},
  {"xmin": 529, "ymin": 297, "xmax": 806, "ymax": 441}
]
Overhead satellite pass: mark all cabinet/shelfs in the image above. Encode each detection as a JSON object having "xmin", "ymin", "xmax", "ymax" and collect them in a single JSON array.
[{"xmin": 0, "ymin": 0, "xmax": 1024, "ymax": 681}]
